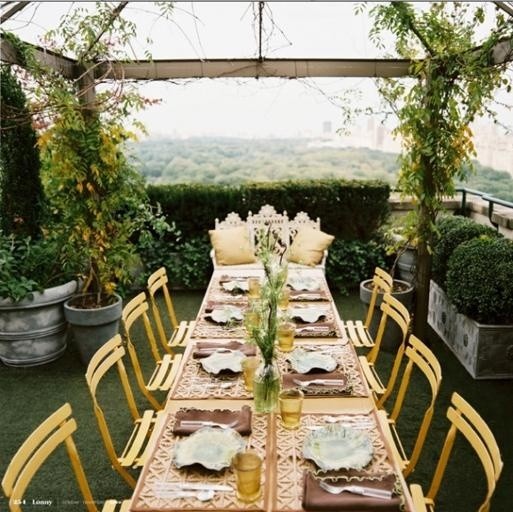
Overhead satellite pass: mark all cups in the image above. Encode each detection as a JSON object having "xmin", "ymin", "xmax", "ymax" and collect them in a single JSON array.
[
  {"xmin": 278, "ymin": 388, "xmax": 303, "ymax": 430},
  {"xmin": 232, "ymin": 452, "xmax": 266, "ymax": 501}
]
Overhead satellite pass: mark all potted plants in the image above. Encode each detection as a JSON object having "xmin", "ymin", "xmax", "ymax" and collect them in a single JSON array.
[
  {"xmin": 0, "ymin": 229, "xmax": 78, "ymax": 369},
  {"xmin": 40, "ymin": 76, "xmax": 154, "ymax": 362},
  {"xmin": 251, "ymin": 224, "xmax": 288, "ymax": 415}
]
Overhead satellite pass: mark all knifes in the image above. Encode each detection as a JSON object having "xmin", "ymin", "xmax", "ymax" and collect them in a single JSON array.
[{"xmin": 154, "ymin": 478, "xmax": 234, "ymax": 491}]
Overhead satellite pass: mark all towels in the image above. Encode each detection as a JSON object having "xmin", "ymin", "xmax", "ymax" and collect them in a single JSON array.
[
  {"xmin": 300, "ymin": 465, "xmax": 403, "ymax": 510},
  {"xmin": 203, "ymin": 299, "xmax": 247, "ymax": 316},
  {"xmin": 293, "ymin": 321, "xmax": 341, "ymax": 339},
  {"xmin": 170, "ymin": 403, "xmax": 252, "ymax": 435},
  {"xmin": 191, "ymin": 337, "xmax": 257, "ymax": 358},
  {"xmin": 287, "ymin": 288, "xmax": 330, "ymax": 302},
  {"xmin": 276, "ymin": 368, "xmax": 353, "ymax": 395},
  {"xmin": 217, "ymin": 273, "xmax": 261, "ymax": 285}
]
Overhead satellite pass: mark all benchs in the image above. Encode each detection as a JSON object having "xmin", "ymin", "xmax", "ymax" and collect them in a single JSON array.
[{"xmin": 207, "ymin": 205, "xmax": 328, "ymax": 275}]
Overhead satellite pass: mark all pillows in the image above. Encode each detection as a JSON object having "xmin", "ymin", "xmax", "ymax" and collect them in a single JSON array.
[
  {"xmin": 208, "ymin": 224, "xmax": 255, "ymax": 265},
  {"xmin": 289, "ymin": 225, "xmax": 336, "ymax": 267}
]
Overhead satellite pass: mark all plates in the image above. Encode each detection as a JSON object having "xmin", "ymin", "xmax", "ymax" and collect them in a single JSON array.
[
  {"xmin": 173, "ymin": 426, "xmax": 246, "ymax": 473},
  {"xmin": 303, "ymin": 425, "xmax": 373, "ymax": 470},
  {"xmin": 201, "ymin": 274, "xmax": 337, "ymax": 391}
]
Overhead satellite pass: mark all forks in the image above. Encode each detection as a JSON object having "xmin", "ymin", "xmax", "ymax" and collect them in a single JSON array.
[
  {"xmin": 291, "ymin": 376, "xmax": 344, "ymax": 389},
  {"xmin": 319, "ymin": 479, "xmax": 391, "ymax": 502},
  {"xmin": 179, "ymin": 419, "xmax": 241, "ymax": 429}
]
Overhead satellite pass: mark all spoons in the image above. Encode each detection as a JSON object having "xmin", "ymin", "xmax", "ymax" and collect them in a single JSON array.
[{"xmin": 152, "ymin": 491, "xmax": 214, "ymax": 503}]
